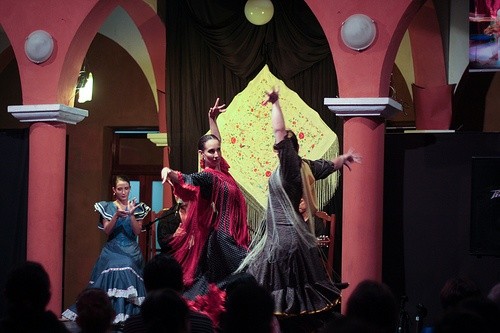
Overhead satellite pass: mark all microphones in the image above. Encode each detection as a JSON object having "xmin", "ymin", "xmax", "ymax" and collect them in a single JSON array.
[{"xmin": 175, "ymin": 198, "xmax": 183, "ymax": 216}]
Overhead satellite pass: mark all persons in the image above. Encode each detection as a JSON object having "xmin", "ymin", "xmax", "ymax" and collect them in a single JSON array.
[
  {"xmin": 322, "ymin": 279, "xmax": 399, "ymax": 333},
  {"xmin": 61, "ymin": 175, "xmax": 152, "ymax": 323},
  {"xmin": 156, "ymin": 197, "xmax": 190, "ymax": 250},
  {"xmin": 433, "ymin": 280, "xmax": 500, "ymax": 333},
  {"xmin": 161, "ymin": 97, "xmax": 250, "ymax": 283},
  {"xmin": 0, "ymin": 255, "xmax": 276, "ymax": 333},
  {"xmin": 236, "ymin": 87, "xmax": 353, "ymax": 333},
  {"xmin": 298, "ymin": 199, "xmax": 324, "ymax": 238}
]
